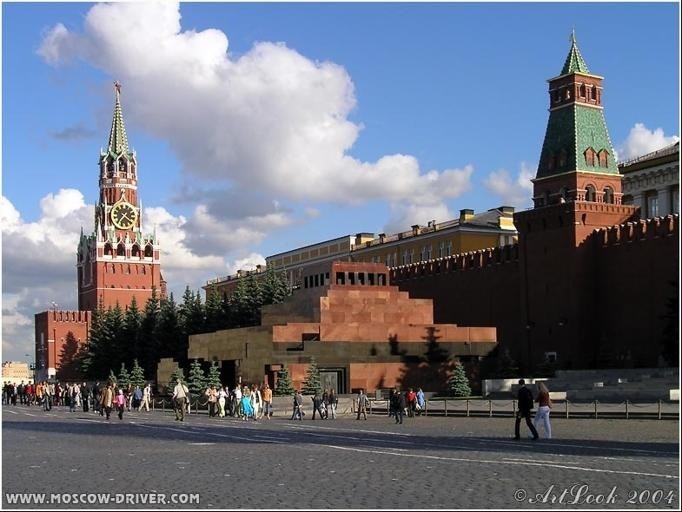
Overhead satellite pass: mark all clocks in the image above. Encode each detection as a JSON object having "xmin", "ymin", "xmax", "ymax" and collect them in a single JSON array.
[{"xmin": 108, "ymin": 202, "xmax": 140, "ymax": 231}]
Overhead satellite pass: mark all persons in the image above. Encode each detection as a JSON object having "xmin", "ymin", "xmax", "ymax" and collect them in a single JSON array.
[
  {"xmin": 528, "ymin": 381, "xmax": 553, "ymax": 440},
  {"xmin": 510, "ymin": 378, "xmax": 540, "ymax": 441}
]
